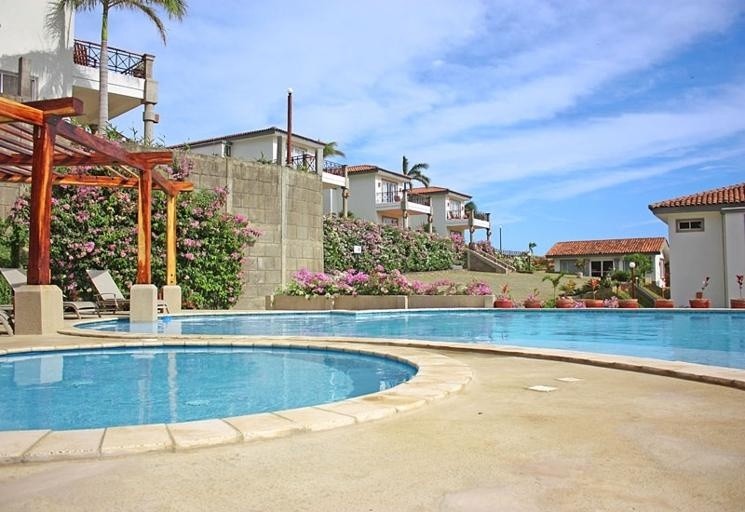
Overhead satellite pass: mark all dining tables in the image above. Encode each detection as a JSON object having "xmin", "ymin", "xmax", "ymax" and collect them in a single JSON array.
[{"xmin": 494, "ymin": 274, "xmax": 745, "ymax": 308}]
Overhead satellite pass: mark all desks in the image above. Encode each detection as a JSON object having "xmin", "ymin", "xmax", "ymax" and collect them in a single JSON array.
[{"xmin": 629, "ymin": 262, "xmax": 636, "ymax": 299}]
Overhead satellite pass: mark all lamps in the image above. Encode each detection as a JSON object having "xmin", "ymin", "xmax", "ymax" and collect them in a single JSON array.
[
  {"xmin": 0, "ymin": 267, "xmax": 102, "ymax": 319},
  {"xmin": 85, "ymin": 270, "xmax": 170, "ymax": 314},
  {"xmin": 74, "ymin": 43, "xmax": 97, "ymax": 68}
]
